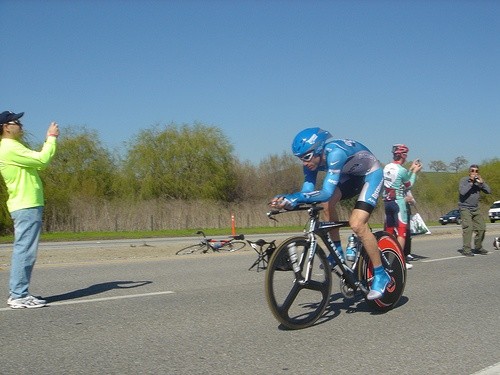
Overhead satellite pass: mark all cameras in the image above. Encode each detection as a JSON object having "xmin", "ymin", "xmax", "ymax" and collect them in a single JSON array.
[{"xmin": 475, "ymin": 176, "xmax": 479, "ymax": 180}]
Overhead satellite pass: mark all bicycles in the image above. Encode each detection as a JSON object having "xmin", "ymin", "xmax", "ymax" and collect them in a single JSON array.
[
  {"xmin": 175, "ymin": 230, "xmax": 247, "ymax": 256},
  {"xmin": 264, "ymin": 200, "xmax": 407, "ymax": 330}
]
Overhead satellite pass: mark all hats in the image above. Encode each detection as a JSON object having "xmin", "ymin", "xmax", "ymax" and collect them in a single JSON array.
[{"xmin": 0, "ymin": 111, "xmax": 24, "ymax": 126}]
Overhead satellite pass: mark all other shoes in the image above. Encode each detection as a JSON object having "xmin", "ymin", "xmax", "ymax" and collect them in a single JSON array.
[
  {"xmin": 462, "ymin": 251, "xmax": 474, "ymax": 257},
  {"xmin": 405, "ymin": 263, "xmax": 412, "ymax": 269},
  {"xmin": 475, "ymin": 249, "xmax": 488, "ymax": 254},
  {"xmin": 406, "ymin": 255, "xmax": 414, "ymax": 261}
]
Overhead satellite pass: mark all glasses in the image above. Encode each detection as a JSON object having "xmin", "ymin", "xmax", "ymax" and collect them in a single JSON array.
[
  {"xmin": 470, "ymin": 169, "xmax": 478, "ymax": 173},
  {"xmin": 300, "ymin": 151, "xmax": 314, "ymax": 162},
  {"xmin": 2, "ymin": 120, "xmax": 20, "ymax": 126}
]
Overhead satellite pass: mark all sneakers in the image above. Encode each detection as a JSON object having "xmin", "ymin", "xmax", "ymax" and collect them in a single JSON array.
[
  {"xmin": 366, "ymin": 270, "xmax": 392, "ymax": 300},
  {"xmin": 7, "ymin": 292, "xmax": 46, "ymax": 309},
  {"xmin": 319, "ymin": 249, "xmax": 345, "ymax": 269}
]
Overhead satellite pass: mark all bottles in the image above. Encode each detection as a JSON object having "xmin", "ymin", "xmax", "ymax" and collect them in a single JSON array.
[{"xmin": 346, "ymin": 234, "xmax": 358, "ymax": 261}]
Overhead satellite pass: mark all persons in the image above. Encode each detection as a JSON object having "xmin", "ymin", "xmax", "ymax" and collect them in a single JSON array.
[
  {"xmin": 382, "ymin": 144, "xmax": 422, "ymax": 270},
  {"xmin": 271, "ymin": 127, "xmax": 391, "ymax": 300},
  {"xmin": 459, "ymin": 165, "xmax": 492, "ymax": 256},
  {"xmin": 1, "ymin": 111, "xmax": 59, "ymax": 308}
]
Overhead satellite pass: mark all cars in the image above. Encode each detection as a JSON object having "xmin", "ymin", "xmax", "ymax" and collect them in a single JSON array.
[{"xmin": 438, "ymin": 209, "xmax": 462, "ymax": 225}]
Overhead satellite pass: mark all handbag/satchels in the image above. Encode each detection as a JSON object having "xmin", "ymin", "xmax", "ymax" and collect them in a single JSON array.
[{"xmin": 408, "ymin": 202, "xmax": 432, "ymax": 234}]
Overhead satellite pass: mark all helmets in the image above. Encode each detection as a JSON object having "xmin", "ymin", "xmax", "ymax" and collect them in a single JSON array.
[
  {"xmin": 392, "ymin": 144, "xmax": 408, "ymax": 158},
  {"xmin": 292, "ymin": 127, "xmax": 325, "ymax": 157}
]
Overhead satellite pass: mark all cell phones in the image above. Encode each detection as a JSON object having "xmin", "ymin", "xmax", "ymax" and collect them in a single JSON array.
[{"xmin": 416, "ymin": 160, "xmax": 419, "ymax": 165}]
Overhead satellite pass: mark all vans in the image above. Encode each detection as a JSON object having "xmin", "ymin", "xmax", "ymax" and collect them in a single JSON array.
[{"xmin": 488, "ymin": 200, "xmax": 500, "ymax": 223}]
